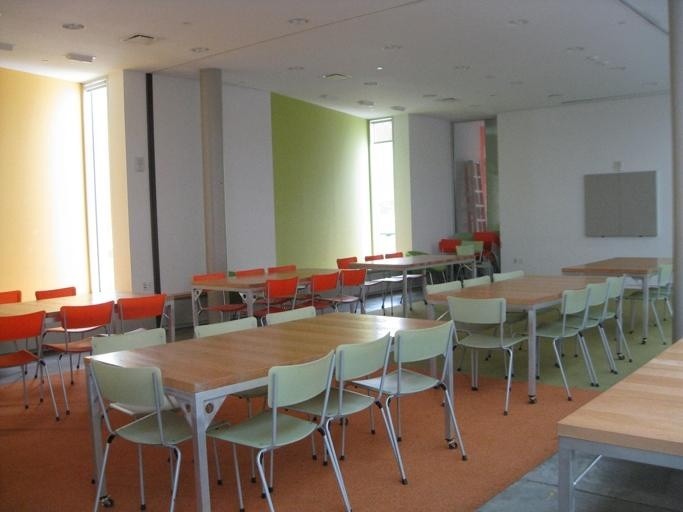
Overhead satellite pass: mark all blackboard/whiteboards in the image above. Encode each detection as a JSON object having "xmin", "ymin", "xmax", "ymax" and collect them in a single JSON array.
[{"xmin": 583, "ymin": 170, "xmax": 657, "ymax": 237}]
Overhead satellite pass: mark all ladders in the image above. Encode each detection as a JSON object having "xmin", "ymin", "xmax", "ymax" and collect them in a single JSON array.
[{"xmin": 467, "ymin": 160, "xmax": 487, "ymax": 232}]
